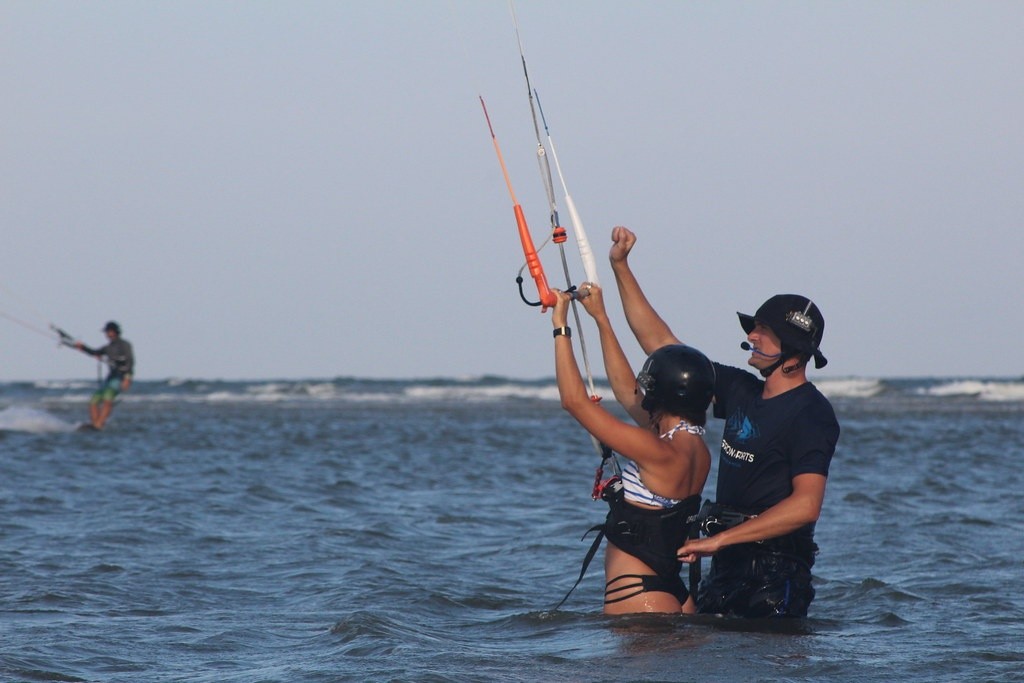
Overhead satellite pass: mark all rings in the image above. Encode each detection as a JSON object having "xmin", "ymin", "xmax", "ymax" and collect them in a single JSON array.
[{"xmin": 587, "ymin": 285, "xmax": 592, "ymax": 289}]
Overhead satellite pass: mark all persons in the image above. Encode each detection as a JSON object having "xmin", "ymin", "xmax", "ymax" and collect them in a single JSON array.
[
  {"xmin": 552, "ymin": 283, "xmax": 716, "ymax": 614},
  {"xmin": 609, "ymin": 227, "xmax": 841, "ymax": 617},
  {"xmin": 77, "ymin": 322, "xmax": 134, "ymax": 430}
]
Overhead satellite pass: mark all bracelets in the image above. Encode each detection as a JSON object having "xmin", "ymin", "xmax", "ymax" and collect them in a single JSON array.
[{"xmin": 553, "ymin": 327, "xmax": 571, "ymax": 337}]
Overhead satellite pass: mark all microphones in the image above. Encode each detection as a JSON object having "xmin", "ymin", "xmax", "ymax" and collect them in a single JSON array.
[{"xmin": 740, "ymin": 341, "xmax": 784, "ymax": 358}]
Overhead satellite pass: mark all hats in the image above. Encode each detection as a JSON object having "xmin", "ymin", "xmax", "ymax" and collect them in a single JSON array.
[{"xmin": 103, "ymin": 321, "xmax": 121, "ymax": 333}]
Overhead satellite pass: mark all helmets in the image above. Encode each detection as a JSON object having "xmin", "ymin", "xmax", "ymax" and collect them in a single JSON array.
[
  {"xmin": 736, "ymin": 294, "xmax": 825, "ymax": 369},
  {"xmin": 634, "ymin": 344, "xmax": 716, "ymax": 428}
]
